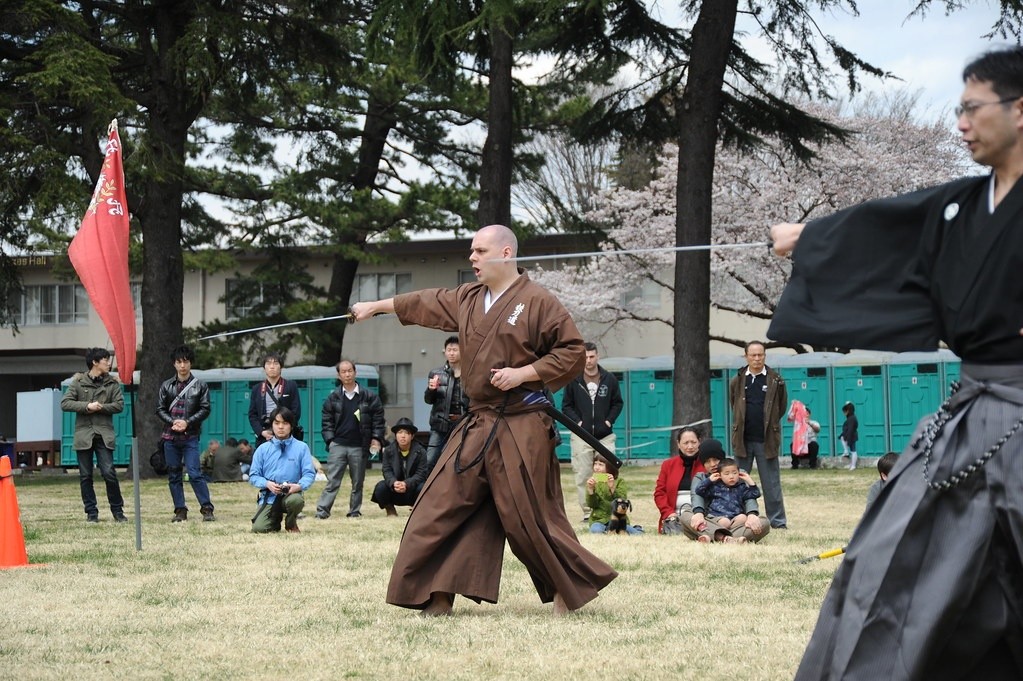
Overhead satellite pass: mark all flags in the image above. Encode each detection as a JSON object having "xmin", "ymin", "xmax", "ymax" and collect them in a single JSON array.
[{"xmin": 67, "ymin": 119, "xmax": 138, "ymax": 384}]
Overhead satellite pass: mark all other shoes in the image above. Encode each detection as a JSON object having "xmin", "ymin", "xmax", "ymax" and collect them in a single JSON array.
[
  {"xmin": 841, "ymin": 454, "xmax": 849, "ymax": 457},
  {"xmin": 386, "ymin": 508, "xmax": 398, "ymax": 517},
  {"xmin": 346, "ymin": 511, "xmax": 362, "ymax": 517},
  {"xmin": 583, "ymin": 514, "xmax": 590, "ymax": 520},
  {"xmin": 115, "ymin": 514, "xmax": 128, "ymax": 522},
  {"xmin": 724, "ymin": 536, "xmax": 746, "ymax": 545},
  {"xmin": 698, "ymin": 535, "xmax": 710, "ymax": 543},
  {"xmin": 315, "ymin": 514, "xmax": 328, "ymax": 519},
  {"xmin": 285, "ymin": 524, "xmax": 301, "ymax": 533},
  {"xmin": 790, "ymin": 466, "xmax": 798, "ymax": 469},
  {"xmin": 633, "ymin": 524, "xmax": 642, "ymax": 529},
  {"xmin": 810, "ymin": 466, "xmax": 816, "ymax": 470},
  {"xmin": 849, "ymin": 467, "xmax": 856, "ymax": 470},
  {"xmin": 88, "ymin": 516, "xmax": 99, "ymax": 523}
]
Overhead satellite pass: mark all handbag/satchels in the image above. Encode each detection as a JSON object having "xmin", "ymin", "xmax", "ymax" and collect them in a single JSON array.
[
  {"xmin": 662, "ymin": 515, "xmax": 682, "ymax": 535},
  {"xmin": 293, "ymin": 426, "xmax": 304, "ymax": 441}
]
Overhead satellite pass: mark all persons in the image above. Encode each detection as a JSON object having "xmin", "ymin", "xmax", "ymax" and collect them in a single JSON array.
[
  {"xmin": 350, "ymin": 225, "xmax": 619, "ymax": 618},
  {"xmin": 654, "ymin": 427, "xmax": 770, "ymax": 545},
  {"xmin": 249, "ymin": 406, "xmax": 316, "ymax": 534},
  {"xmin": 156, "ymin": 346, "xmax": 213, "ymax": 522},
  {"xmin": 730, "ymin": 341, "xmax": 787, "ymax": 530},
  {"xmin": 371, "ymin": 418, "xmax": 429, "ymax": 515},
  {"xmin": 586, "ymin": 454, "xmax": 644, "ymax": 535},
  {"xmin": 61, "ymin": 348, "xmax": 127, "ymax": 522},
  {"xmin": 562, "ymin": 342, "xmax": 624, "ymax": 518},
  {"xmin": 248, "ymin": 351, "xmax": 301, "ymax": 448},
  {"xmin": 866, "ymin": 452, "xmax": 900, "ymax": 505},
  {"xmin": 767, "ymin": 44, "xmax": 1023, "ymax": 681},
  {"xmin": 787, "ymin": 400, "xmax": 820, "ymax": 470},
  {"xmin": 199, "ymin": 437, "xmax": 254, "ymax": 484},
  {"xmin": 838, "ymin": 403, "xmax": 858, "ymax": 470},
  {"xmin": 424, "ymin": 337, "xmax": 470, "ymax": 478},
  {"xmin": 315, "ymin": 361, "xmax": 385, "ymax": 519}
]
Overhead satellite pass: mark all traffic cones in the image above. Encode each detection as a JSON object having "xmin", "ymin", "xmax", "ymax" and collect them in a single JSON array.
[{"xmin": 0, "ymin": 456, "xmax": 46, "ymax": 570}]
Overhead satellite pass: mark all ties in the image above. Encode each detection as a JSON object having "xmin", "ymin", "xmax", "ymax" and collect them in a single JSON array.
[{"xmin": 280, "ymin": 443, "xmax": 286, "ymax": 456}]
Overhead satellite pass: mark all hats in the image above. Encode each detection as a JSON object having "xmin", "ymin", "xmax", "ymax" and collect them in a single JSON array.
[
  {"xmin": 698, "ymin": 439, "xmax": 726, "ymax": 466},
  {"xmin": 391, "ymin": 417, "xmax": 418, "ymax": 434}
]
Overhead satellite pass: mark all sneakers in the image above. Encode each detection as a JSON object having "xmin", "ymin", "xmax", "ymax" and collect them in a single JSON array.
[
  {"xmin": 171, "ymin": 510, "xmax": 187, "ymax": 522},
  {"xmin": 201, "ymin": 510, "xmax": 215, "ymax": 522}
]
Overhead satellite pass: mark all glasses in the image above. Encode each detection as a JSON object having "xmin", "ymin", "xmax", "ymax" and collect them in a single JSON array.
[{"xmin": 954, "ymin": 97, "xmax": 1018, "ymax": 118}]
